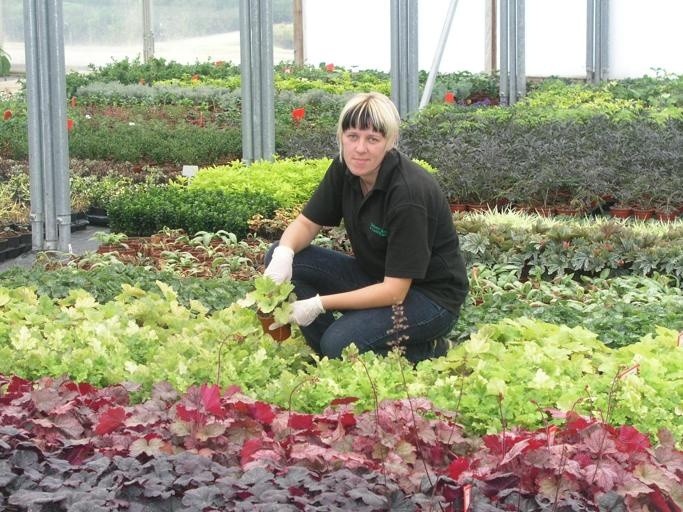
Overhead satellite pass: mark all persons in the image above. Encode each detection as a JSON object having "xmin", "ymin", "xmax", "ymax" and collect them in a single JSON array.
[{"xmin": 264, "ymin": 92, "xmax": 470, "ymax": 372}]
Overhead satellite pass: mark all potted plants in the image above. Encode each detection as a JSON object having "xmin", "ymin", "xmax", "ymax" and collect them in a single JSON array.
[
  {"xmin": 70, "ymin": 174, "xmax": 111, "ymax": 232},
  {"xmin": 0, "ymin": 172, "xmax": 33, "ymax": 264},
  {"xmin": 435, "ymin": 120, "xmax": 683, "ymax": 223}
]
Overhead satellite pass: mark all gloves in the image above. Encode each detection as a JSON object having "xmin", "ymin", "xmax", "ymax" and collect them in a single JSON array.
[
  {"xmin": 262, "ymin": 246, "xmax": 294, "ymax": 285},
  {"xmin": 268, "ymin": 293, "xmax": 327, "ymax": 331}
]
